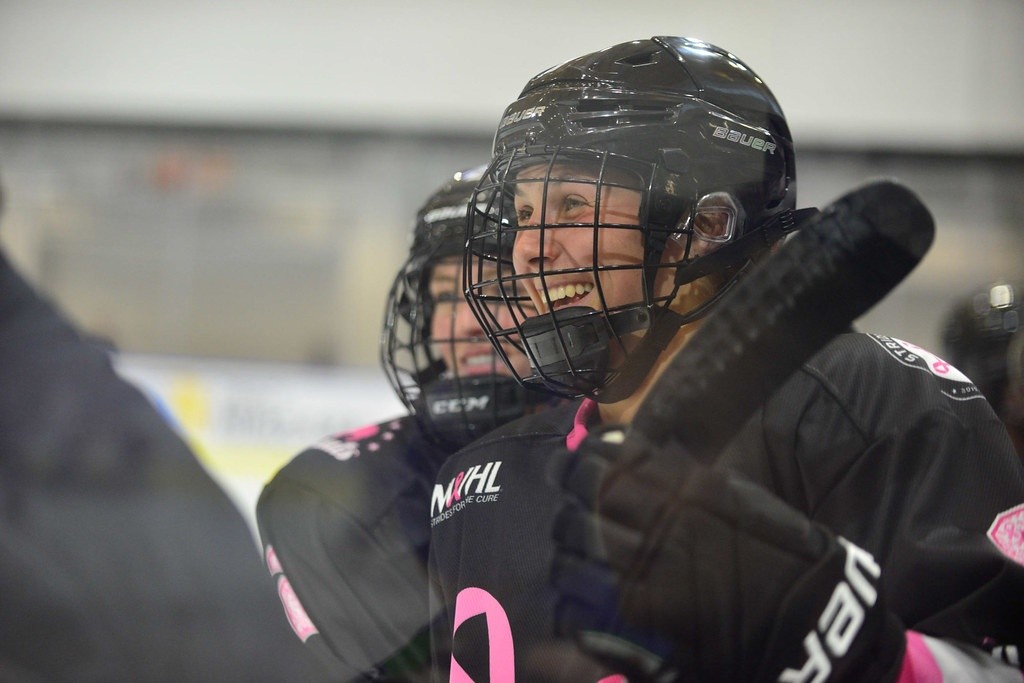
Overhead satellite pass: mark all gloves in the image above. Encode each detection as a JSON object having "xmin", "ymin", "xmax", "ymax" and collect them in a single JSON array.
[{"xmin": 546, "ymin": 422, "xmax": 904, "ymax": 683}]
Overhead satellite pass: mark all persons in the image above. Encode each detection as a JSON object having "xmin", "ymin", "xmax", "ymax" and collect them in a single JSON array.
[
  {"xmin": 1, "ymin": 249, "xmax": 336, "ymax": 682},
  {"xmin": 428, "ymin": 36, "xmax": 1024, "ymax": 683},
  {"xmin": 254, "ymin": 165, "xmax": 573, "ymax": 683}
]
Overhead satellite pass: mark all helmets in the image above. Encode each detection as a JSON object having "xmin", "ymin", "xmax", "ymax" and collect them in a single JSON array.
[
  {"xmin": 380, "ymin": 163, "xmax": 534, "ymax": 451},
  {"xmin": 465, "ymin": 36, "xmax": 797, "ymax": 404}
]
